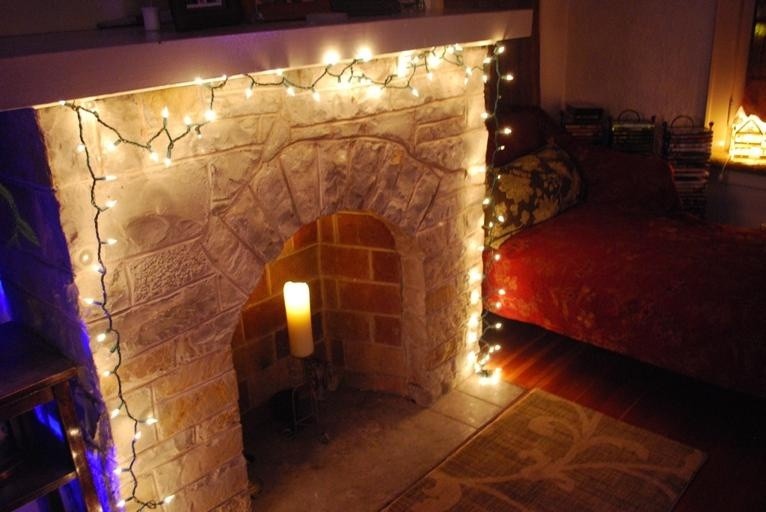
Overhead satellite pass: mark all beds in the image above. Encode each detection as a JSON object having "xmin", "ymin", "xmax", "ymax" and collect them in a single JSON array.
[{"xmin": 480, "ymin": 104, "xmax": 765, "ymax": 398}]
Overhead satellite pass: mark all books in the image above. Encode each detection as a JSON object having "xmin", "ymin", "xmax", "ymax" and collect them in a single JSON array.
[
  {"xmin": 562, "ymin": 118, "xmax": 655, "ymax": 154},
  {"xmin": 667, "ymin": 125, "xmax": 714, "ymax": 220}
]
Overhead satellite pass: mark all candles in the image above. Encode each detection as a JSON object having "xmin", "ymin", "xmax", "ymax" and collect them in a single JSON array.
[{"xmin": 281, "ymin": 280, "xmax": 314, "ymax": 358}]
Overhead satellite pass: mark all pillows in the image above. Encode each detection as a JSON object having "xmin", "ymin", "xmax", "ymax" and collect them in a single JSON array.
[{"xmin": 483, "ymin": 146, "xmax": 581, "ymax": 250}]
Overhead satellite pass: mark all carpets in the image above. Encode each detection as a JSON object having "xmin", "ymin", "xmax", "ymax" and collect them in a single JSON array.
[{"xmin": 375, "ymin": 386, "xmax": 715, "ymax": 512}]
